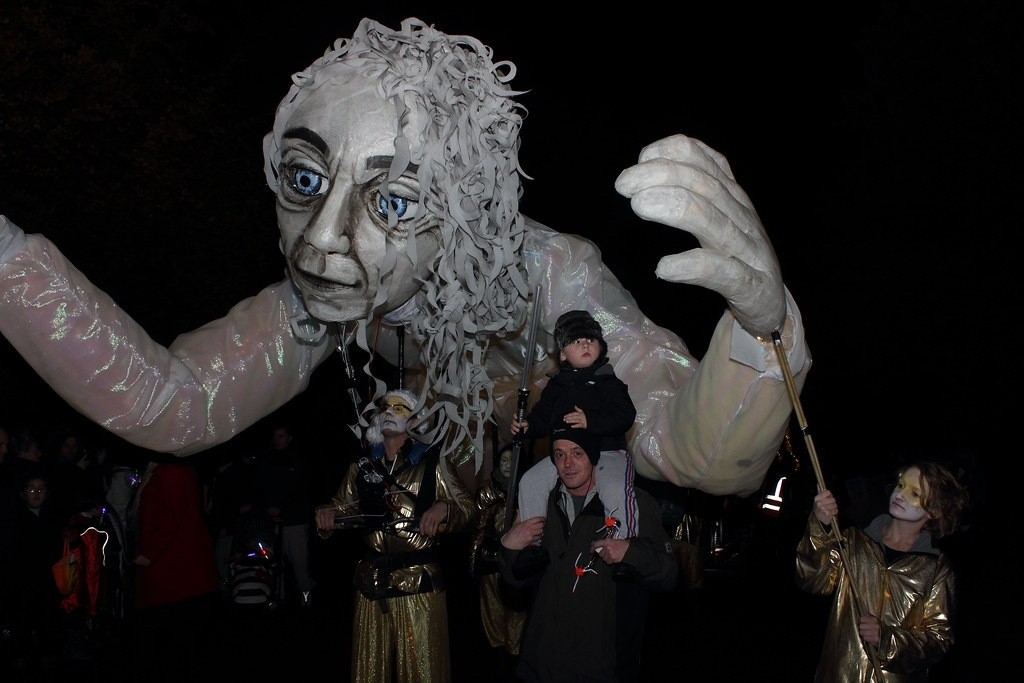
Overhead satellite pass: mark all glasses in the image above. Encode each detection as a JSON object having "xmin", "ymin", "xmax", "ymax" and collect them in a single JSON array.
[
  {"xmin": 382, "ymin": 403, "xmax": 412, "ymax": 415},
  {"xmin": 26, "ymin": 488, "xmax": 47, "ymax": 495}
]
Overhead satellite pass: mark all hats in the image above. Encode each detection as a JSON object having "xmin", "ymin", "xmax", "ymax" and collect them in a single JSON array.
[
  {"xmin": 553, "ymin": 310, "xmax": 608, "ymax": 353},
  {"xmin": 549, "ymin": 421, "xmax": 600, "ymax": 468}
]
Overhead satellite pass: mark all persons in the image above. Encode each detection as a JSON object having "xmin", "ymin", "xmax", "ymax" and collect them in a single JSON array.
[
  {"xmin": 315, "ymin": 386, "xmax": 479, "ymax": 683},
  {"xmin": 0, "ymin": 18, "xmax": 815, "ymax": 498},
  {"xmin": 472, "ymin": 444, "xmax": 530, "ymax": 659},
  {"xmin": 795, "ymin": 462, "xmax": 970, "ymax": 683},
  {"xmin": 497, "ymin": 400, "xmax": 685, "ymax": 683},
  {"xmin": 512, "ymin": 311, "xmax": 641, "ymax": 581},
  {"xmin": 0, "ymin": 404, "xmax": 318, "ymax": 636}
]
